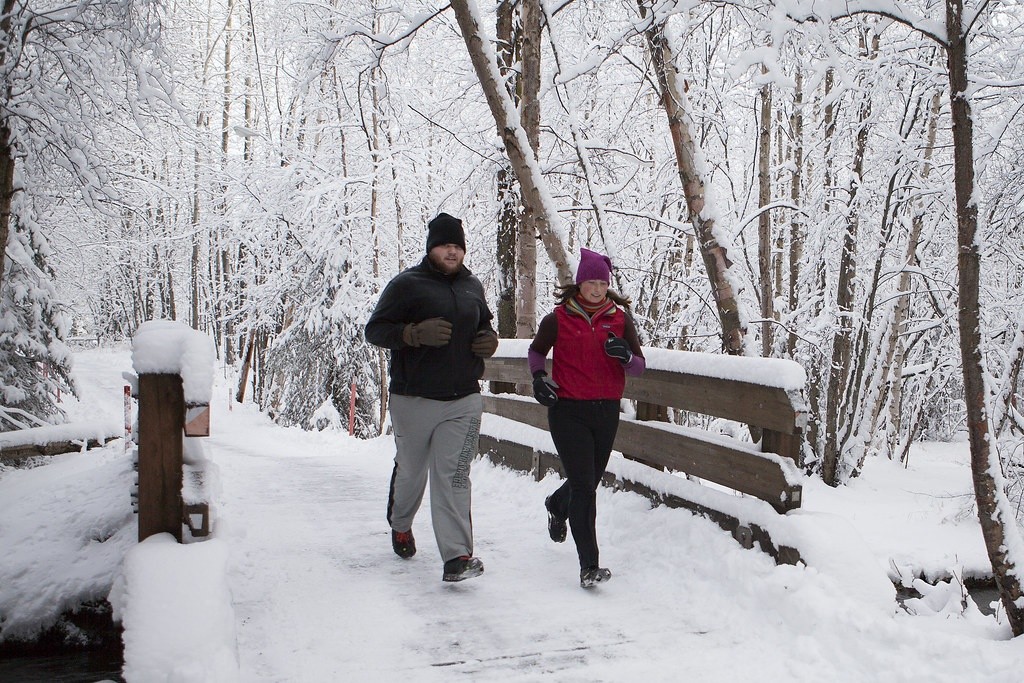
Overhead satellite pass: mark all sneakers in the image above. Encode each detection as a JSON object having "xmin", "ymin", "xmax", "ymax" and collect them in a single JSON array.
[
  {"xmin": 443, "ymin": 556, "xmax": 484, "ymax": 581},
  {"xmin": 545, "ymin": 496, "xmax": 567, "ymax": 543},
  {"xmin": 580, "ymin": 567, "xmax": 611, "ymax": 588},
  {"xmin": 392, "ymin": 527, "xmax": 416, "ymax": 558}
]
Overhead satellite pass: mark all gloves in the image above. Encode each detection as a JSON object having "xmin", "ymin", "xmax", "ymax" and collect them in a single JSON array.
[
  {"xmin": 471, "ymin": 330, "xmax": 498, "ymax": 358},
  {"xmin": 604, "ymin": 331, "xmax": 632, "ymax": 366},
  {"xmin": 402, "ymin": 317, "xmax": 452, "ymax": 348},
  {"xmin": 532, "ymin": 375, "xmax": 559, "ymax": 407}
]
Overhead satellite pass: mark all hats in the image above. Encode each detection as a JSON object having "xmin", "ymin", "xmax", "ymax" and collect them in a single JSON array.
[
  {"xmin": 576, "ymin": 248, "xmax": 612, "ymax": 287},
  {"xmin": 427, "ymin": 213, "xmax": 466, "ymax": 255}
]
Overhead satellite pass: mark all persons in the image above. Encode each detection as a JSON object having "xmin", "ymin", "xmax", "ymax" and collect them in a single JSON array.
[
  {"xmin": 528, "ymin": 248, "xmax": 646, "ymax": 588},
  {"xmin": 364, "ymin": 212, "xmax": 498, "ymax": 582}
]
